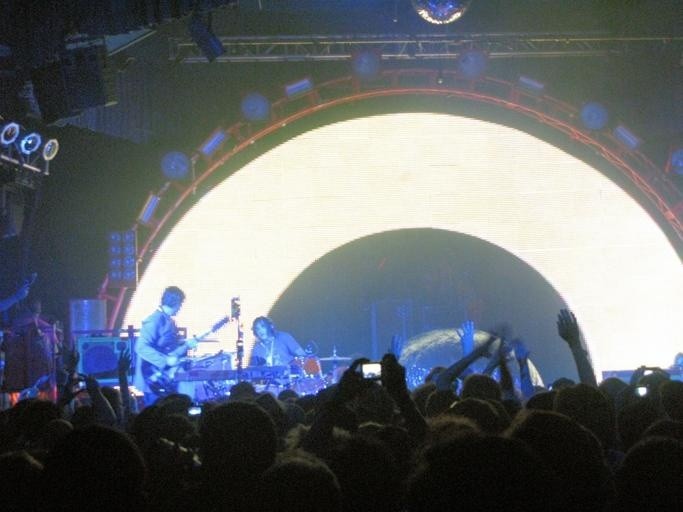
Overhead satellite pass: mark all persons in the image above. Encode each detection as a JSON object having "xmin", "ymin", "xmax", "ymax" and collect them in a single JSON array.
[
  {"xmin": 133, "ymin": 287, "xmax": 195, "ymax": 405},
  {"xmin": 0, "ymin": 284, "xmax": 30, "ymax": 310},
  {"xmin": 0, "ymin": 309, "xmax": 682, "ymax": 509}
]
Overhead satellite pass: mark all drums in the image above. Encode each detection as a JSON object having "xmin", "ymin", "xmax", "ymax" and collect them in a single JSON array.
[
  {"xmin": 288, "ymin": 356, "xmax": 318, "ymax": 375},
  {"xmin": 291, "ymin": 376, "xmax": 324, "ymax": 397},
  {"xmin": 325, "ymin": 366, "xmax": 351, "ymax": 387}
]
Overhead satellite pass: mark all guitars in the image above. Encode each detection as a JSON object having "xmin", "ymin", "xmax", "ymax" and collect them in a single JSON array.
[{"xmin": 141, "ymin": 315, "xmax": 230, "ymax": 393}]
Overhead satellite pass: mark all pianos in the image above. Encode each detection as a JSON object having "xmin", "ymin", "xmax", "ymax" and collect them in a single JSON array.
[{"xmin": 173, "ymin": 365, "xmax": 288, "ymax": 382}]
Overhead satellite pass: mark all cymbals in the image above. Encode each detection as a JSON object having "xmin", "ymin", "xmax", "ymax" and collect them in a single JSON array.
[{"xmin": 321, "ymin": 356, "xmax": 351, "ymax": 361}]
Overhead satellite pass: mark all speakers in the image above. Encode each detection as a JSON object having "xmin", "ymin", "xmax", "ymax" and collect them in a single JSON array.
[{"xmin": 76, "ymin": 337, "xmax": 133, "ymax": 387}]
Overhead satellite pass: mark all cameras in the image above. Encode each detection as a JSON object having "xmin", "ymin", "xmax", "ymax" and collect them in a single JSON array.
[
  {"xmin": 633, "ymin": 385, "xmax": 650, "ymax": 397},
  {"xmin": 360, "ymin": 362, "xmax": 383, "ymax": 380},
  {"xmin": 642, "ymin": 368, "xmax": 657, "ymax": 377},
  {"xmin": 186, "ymin": 406, "xmax": 203, "ymax": 417}
]
